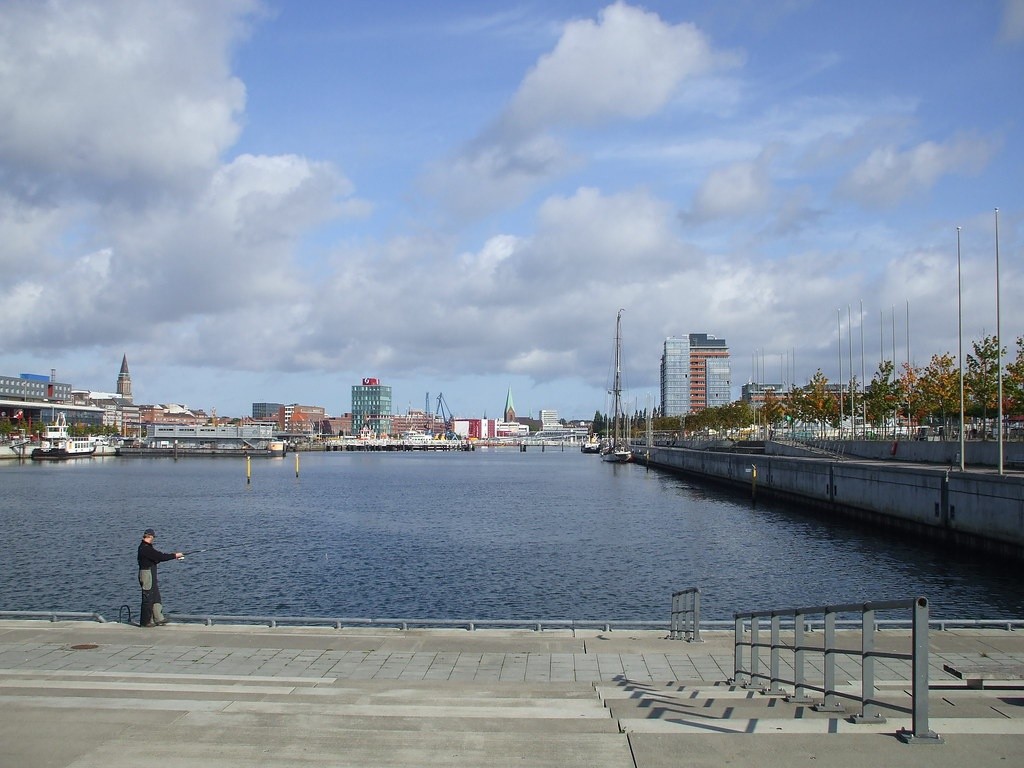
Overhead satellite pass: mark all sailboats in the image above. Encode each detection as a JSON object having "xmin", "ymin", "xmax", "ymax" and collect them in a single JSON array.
[{"xmin": 601, "ymin": 308, "xmax": 632, "ymax": 462}]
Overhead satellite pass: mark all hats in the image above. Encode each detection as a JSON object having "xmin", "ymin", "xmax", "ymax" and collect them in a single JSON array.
[{"xmin": 144, "ymin": 528, "xmax": 154, "ymax": 535}]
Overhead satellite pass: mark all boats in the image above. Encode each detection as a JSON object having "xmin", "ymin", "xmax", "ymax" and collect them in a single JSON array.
[
  {"xmin": 581, "ymin": 435, "xmax": 601, "ymax": 454},
  {"xmin": 31, "ymin": 411, "xmax": 124, "ymax": 459}
]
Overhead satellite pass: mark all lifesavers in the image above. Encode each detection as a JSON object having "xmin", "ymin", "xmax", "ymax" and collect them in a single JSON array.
[{"xmin": 891, "ymin": 443, "xmax": 896, "ymax": 455}]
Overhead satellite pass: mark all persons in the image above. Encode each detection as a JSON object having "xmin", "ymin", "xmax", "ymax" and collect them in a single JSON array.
[{"xmin": 137, "ymin": 528, "xmax": 183, "ymax": 626}]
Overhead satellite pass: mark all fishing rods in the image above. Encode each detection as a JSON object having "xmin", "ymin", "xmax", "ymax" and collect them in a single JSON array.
[{"xmin": 178, "ymin": 542, "xmax": 253, "ymax": 560}]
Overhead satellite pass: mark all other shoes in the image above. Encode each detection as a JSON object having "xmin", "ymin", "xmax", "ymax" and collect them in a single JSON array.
[{"xmin": 140, "ymin": 619, "xmax": 168, "ymax": 627}]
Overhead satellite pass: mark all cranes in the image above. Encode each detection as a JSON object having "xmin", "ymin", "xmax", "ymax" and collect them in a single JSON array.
[{"xmin": 435, "ymin": 392, "xmax": 455, "ymax": 440}]
